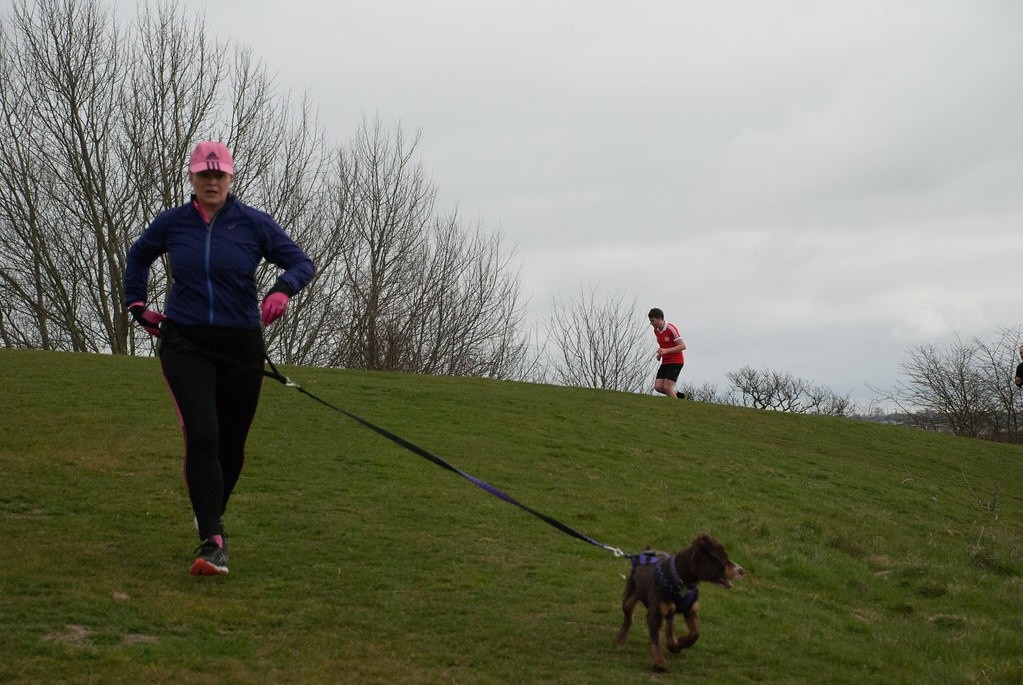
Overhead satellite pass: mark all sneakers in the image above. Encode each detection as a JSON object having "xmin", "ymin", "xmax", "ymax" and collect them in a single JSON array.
[{"xmin": 191, "ymin": 516, "xmax": 231, "ymax": 577}]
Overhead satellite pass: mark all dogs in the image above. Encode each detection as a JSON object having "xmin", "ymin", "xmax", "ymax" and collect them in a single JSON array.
[{"xmin": 612, "ymin": 533, "xmax": 748, "ymax": 673}]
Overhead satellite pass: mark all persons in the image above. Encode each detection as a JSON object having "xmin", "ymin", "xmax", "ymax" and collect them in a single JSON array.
[
  {"xmin": 648, "ymin": 308, "xmax": 686, "ymax": 399},
  {"xmin": 1015, "ymin": 345, "xmax": 1023, "ymax": 388},
  {"xmin": 124, "ymin": 142, "xmax": 316, "ymax": 574}
]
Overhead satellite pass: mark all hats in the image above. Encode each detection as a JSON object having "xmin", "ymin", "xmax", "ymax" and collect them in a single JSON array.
[{"xmin": 189, "ymin": 141, "xmax": 235, "ymax": 177}]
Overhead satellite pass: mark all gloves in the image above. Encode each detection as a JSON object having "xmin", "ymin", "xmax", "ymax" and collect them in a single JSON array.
[
  {"xmin": 259, "ymin": 289, "xmax": 288, "ymax": 326},
  {"xmin": 129, "ymin": 304, "xmax": 166, "ymax": 338}
]
